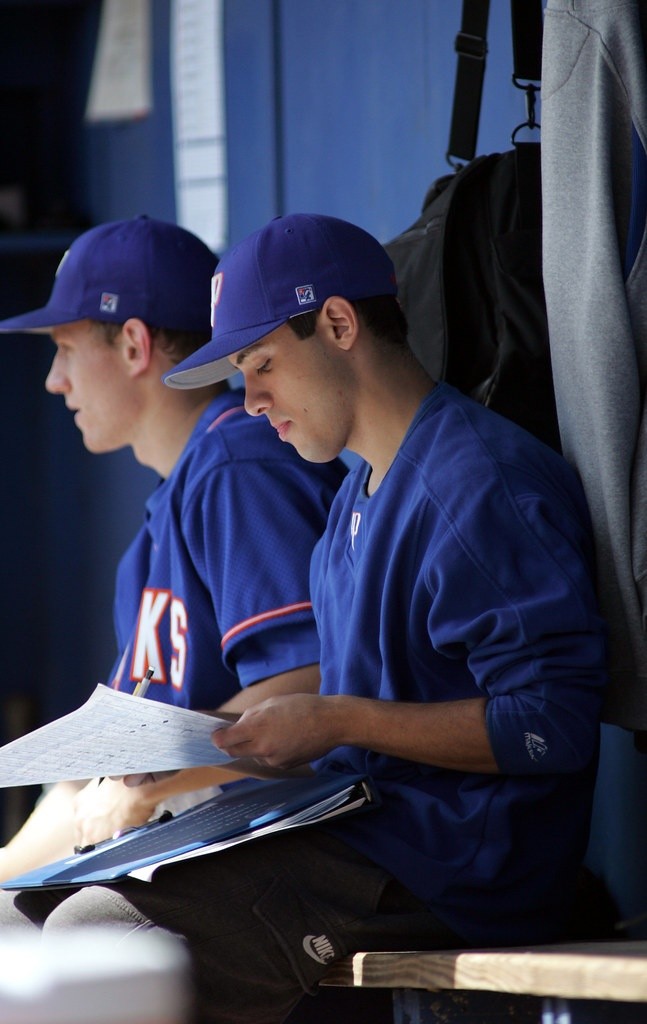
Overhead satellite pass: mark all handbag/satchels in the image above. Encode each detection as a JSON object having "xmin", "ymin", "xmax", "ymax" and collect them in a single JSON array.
[{"xmin": 374, "ymin": 132, "xmax": 559, "ymax": 444}]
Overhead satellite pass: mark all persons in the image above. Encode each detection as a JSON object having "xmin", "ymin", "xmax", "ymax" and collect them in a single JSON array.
[{"xmin": 0, "ymin": 217, "xmax": 603, "ymax": 1024}]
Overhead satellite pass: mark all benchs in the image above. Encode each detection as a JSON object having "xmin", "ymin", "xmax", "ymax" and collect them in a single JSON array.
[{"xmin": 320, "ymin": 937, "xmax": 646, "ymax": 1024}]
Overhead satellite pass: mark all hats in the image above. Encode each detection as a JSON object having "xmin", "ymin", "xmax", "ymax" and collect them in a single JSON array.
[
  {"xmin": 160, "ymin": 214, "xmax": 398, "ymax": 390},
  {"xmin": 0, "ymin": 214, "xmax": 219, "ymax": 336}
]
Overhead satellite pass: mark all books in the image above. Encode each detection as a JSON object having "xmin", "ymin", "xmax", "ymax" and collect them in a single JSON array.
[{"xmin": 2, "ymin": 774, "xmax": 377, "ymax": 891}]
